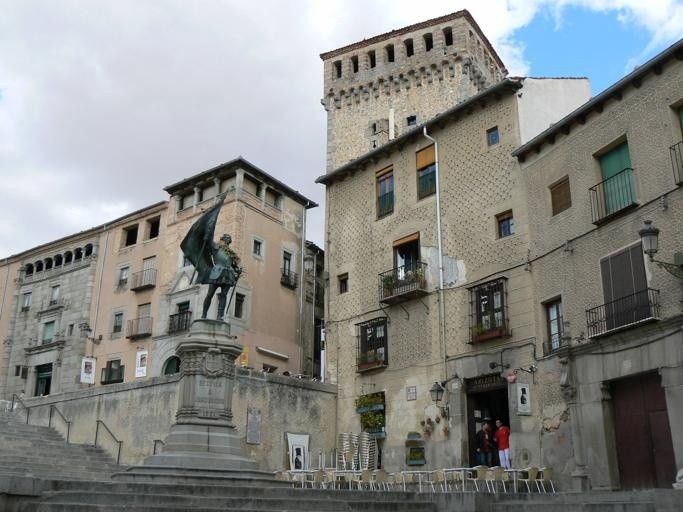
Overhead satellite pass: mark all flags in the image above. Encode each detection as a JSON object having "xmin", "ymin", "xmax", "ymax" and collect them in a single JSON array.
[{"xmin": 180, "ymin": 196, "xmax": 225, "ymax": 284}]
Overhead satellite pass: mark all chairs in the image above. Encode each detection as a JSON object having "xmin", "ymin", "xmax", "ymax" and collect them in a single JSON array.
[{"xmin": 286, "ymin": 464, "xmax": 555, "ymax": 493}]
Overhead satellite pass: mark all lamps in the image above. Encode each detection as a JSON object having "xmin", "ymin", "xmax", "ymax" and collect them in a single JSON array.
[
  {"xmin": 637, "ymin": 219, "xmax": 681, "ymax": 279},
  {"xmin": 428, "ymin": 381, "xmax": 448, "ymax": 410}
]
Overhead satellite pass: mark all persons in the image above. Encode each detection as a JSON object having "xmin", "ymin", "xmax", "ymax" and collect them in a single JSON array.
[
  {"xmin": 473, "ymin": 421, "xmax": 494, "ymax": 467},
  {"xmin": 492, "ymin": 418, "xmax": 511, "ymax": 469},
  {"xmin": 202, "ymin": 233, "xmax": 242, "ymax": 320}
]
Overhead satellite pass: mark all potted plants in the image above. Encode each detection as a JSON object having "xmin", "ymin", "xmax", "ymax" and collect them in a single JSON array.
[
  {"xmin": 357, "ymin": 354, "xmax": 385, "ymax": 369},
  {"xmin": 469, "ymin": 315, "xmax": 504, "ymax": 340},
  {"xmin": 383, "ymin": 268, "xmax": 424, "ymax": 296}
]
